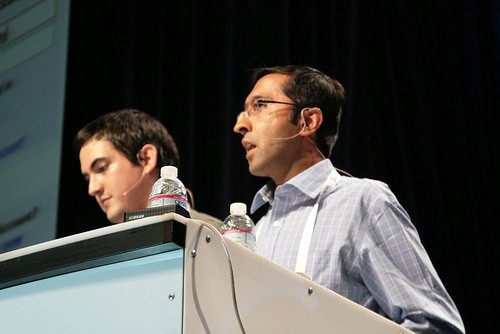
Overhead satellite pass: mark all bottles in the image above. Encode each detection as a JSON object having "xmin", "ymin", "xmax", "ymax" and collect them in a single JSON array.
[
  {"xmin": 148, "ymin": 165, "xmax": 190, "ymax": 212},
  {"xmin": 219, "ymin": 202, "xmax": 258, "ymax": 252}
]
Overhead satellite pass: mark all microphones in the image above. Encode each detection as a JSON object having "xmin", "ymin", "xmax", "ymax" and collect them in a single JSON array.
[
  {"xmin": 123, "ymin": 153, "xmax": 145, "ymax": 196},
  {"xmin": 271, "ymin": 107, "xmax": 310, "ymax": 140}
]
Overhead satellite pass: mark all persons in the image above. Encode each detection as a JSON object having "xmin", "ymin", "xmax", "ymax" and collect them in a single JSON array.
[
  {"xmin": 72, "ymin": 108, "xmax": 224, "ymax": 233},
  {"xmin": 232, "ymin": 65, "xmax": 467, "ymax": 334}
]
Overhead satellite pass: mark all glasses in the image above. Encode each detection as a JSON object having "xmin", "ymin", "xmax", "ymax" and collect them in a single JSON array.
[{"xmin": 244, "ymin": 98, "xmax": 300, "ymax": 121}]
